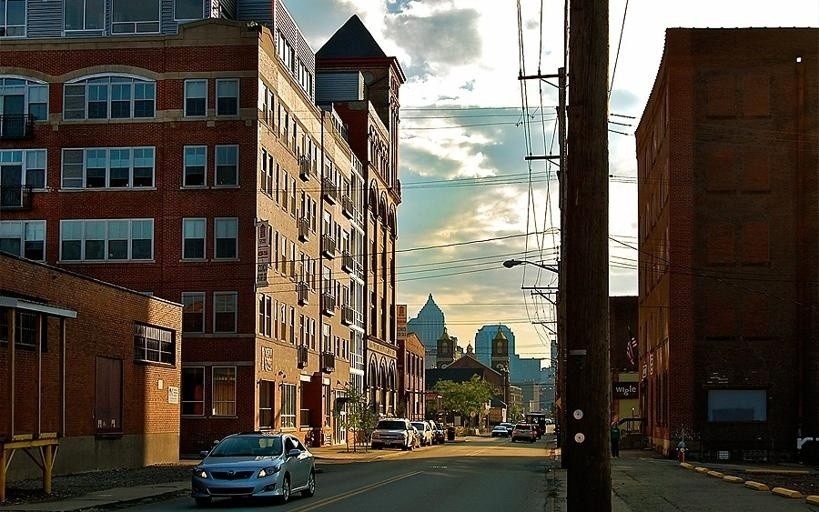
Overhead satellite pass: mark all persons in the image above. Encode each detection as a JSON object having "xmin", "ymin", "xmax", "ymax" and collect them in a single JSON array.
[{"xmin": 609, "ymin": 414, "xmax": 620, "ymax": 458}]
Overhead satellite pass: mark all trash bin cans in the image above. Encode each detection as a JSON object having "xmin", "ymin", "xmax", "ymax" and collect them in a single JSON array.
[{"xmin": 447, "ymin": 426, "xmax": 456, "ymax": 441}]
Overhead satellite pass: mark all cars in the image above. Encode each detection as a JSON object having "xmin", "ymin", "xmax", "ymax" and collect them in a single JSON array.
[
  {"xmin": 490, "ymin": 411, "xmax": 556, "ymax": 445},
  {"xmin": 614, "ymin": 416, "xmax": 642, "ymax": 451},
  {"xmin": 189, "ymin": 428, "xmax": 317, "ymax": 508},
  {"xmin": 371, "ymin": 416, "xmax": 448, "ymax": 451}
]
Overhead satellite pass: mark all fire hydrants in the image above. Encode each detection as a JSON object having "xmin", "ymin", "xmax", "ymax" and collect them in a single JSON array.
[{"xmin": 676, "ymin": 441, "xmax": 689, "ymax": 463}]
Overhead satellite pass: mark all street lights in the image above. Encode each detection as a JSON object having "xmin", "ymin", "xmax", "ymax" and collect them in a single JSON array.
[{"xmin": 502, "ymin": 256, "xmax": 567, "ymax": 448}]
